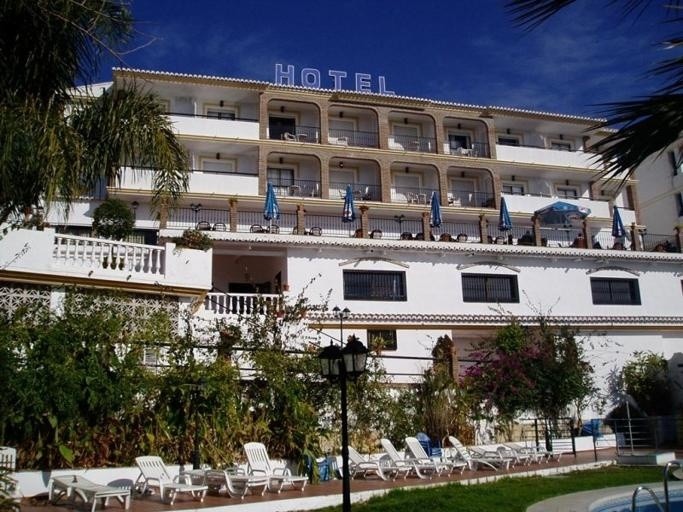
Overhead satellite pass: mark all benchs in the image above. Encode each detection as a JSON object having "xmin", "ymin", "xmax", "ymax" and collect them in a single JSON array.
[{"xmin": 618, "ymin": 451, "xmax": 674, "ymax": 466}]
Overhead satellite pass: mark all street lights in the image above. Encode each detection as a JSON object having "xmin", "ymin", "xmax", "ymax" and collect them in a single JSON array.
[{"xmin": 319, "ymin": 335, "xmax": 367, "ymax": 510}]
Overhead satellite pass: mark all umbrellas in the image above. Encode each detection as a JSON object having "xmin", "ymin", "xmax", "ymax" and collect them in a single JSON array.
[
  {"xmin": 427, "ymin": 191, "xmax": 441, "ymax": 239},
  {"xmin": 340, "ymin": 184, "xmax": 356, "ymax": 237},
  {"xmin": 262, "ymin": 181, "xmax": 279, "ymax": 233},
  {"xmin": 532, "ymin": 200, "xmax": 590, "ymax": 247},
  {"xmin": 611, "ymin": 205, "xmax": 626, "ymax": 238},
  {"xmin": 497, "ymin": 196, "xmax": 511, "ymax": 245}
]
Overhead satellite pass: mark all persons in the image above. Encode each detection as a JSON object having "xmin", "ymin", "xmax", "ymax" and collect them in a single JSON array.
[
  {"xmin": 613, "ymin": 242, "xmax": 621, "ymax": 249},
  {"xmin": 591, "ymin": 235, "xmax": 596, "ymax": 247},
  {"xmin": 654, "ymin": 243, "xmax": 666, "ymax": 252},
  {"xmin": 519, "ymin": 230, "xmax": 531, "ymax": 242}
]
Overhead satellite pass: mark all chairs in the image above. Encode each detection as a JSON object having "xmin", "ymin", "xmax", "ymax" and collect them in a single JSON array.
[
  {"xmin": 407, "ymin": 192, "xmax": 419, "ymax": 203},
  {"xmin": 284, "ymin": 132, "xmax": 308, "ymax": 141},
  {"xmin": 336, "ymin": 137, "xmax": 348, "ymax": 145}
]
